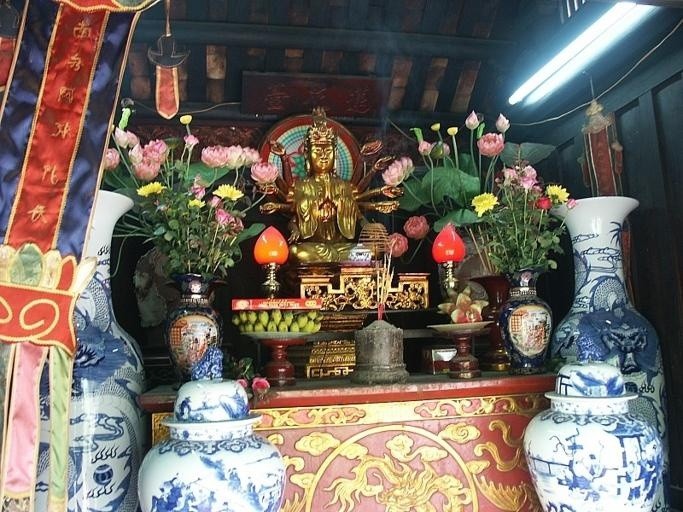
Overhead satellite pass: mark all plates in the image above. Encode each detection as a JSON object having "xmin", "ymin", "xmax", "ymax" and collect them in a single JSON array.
[
  {"xmin": 238, "ymin": 332, "xmax": 314, "ymax": 339},
  {"xmin": 426, "ymin": 321, "xmax": 495, "ymax": 334}
]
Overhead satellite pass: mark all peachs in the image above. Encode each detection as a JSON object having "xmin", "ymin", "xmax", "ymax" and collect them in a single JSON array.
[
  {"xmin": 465, "ymin": 308, "xmax": 481, "ymax": 322},
  {"xmin": 456, "ymin": 293, "xmax": 472, "ymax": 312},
  {"xmin": 450, "ymin": 309, "xmax": 467, "ymax": 324}
]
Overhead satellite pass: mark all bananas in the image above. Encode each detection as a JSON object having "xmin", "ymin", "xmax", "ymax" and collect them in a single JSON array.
[{"xmin": 231, "ymin": 309, "xmax": 324, "ymax": 335}]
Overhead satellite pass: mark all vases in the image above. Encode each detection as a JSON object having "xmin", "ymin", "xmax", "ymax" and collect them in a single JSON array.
[
  {"xmin": 468, "ymin": 274, "xmax": 511, "ymax": 372},
  {"xmin": 548, "ymin": 194, "xmax": 674, "ymax": 512},
  {"xmin": 495, "ymin": 267, "xmax": 554, "ymax": 376},
  {"xmin": 162, "ymin": 271, "xmax": 225, "ymax": 392},
  {"xmin": 29, "ymin": 188, "xmax": 148, "ymax": 511}
]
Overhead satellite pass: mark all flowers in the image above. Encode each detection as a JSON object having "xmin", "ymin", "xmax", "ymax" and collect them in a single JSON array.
[
  {"xmin": 470, "ymin": 164, "xmax": 578, "ymax": 273},
  {"xmin": 381, "ymin": 110, "xmax": 558, "ymax": 275},
  {"xmin": 101, "ymin": 114, "xmax": 279, "ymax": 280}
]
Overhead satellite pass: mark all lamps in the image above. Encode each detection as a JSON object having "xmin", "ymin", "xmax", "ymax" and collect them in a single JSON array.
[
  {"xmin": 523, "ymin": 2, "xmax": 665, "ymax": 114},
  {"xmin": 507, "ymin": 0, "xmax": 637, "ymax": 106}
]
